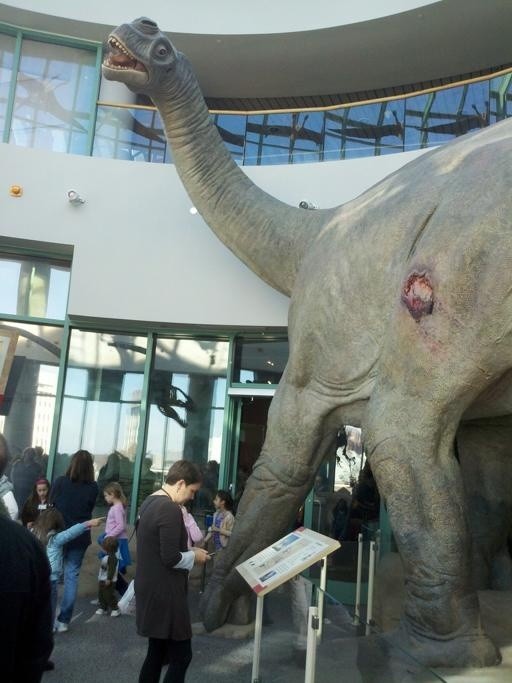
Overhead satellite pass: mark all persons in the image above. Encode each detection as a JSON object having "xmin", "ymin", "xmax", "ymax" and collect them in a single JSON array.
[
  {"xmin": 31, "ymin": 508, "xmax": 106, "ymax": 620},
  {"xmin": 0, "ymin": 434, "xmax": 98, "ymax": 683},
  {"xmin": 90, "ymin": 482, "xmax": 132, "ymax": 617},
  {"xmin": 135, "ymin": 460, "xmax": 210, "ymax": 683},
  {"xmin": 293, "ymin": 462, "xmax": 383, "ymax": 586},
  {"xmin": 178, "ymin": 489, "xmax": 234, "ymax": 554}
]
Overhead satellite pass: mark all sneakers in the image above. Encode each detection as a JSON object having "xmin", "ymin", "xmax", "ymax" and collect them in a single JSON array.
[
  {"xmin": 111, "ymin": 609, "xmax": 121, "ymax": 616},
  {"xmin": 55, "ymin": 619, "xmax": 68, "ymax": 632},
  {"xmin": 96, "ymin": 608, "xmax": 108, "ymax": 614}
]
